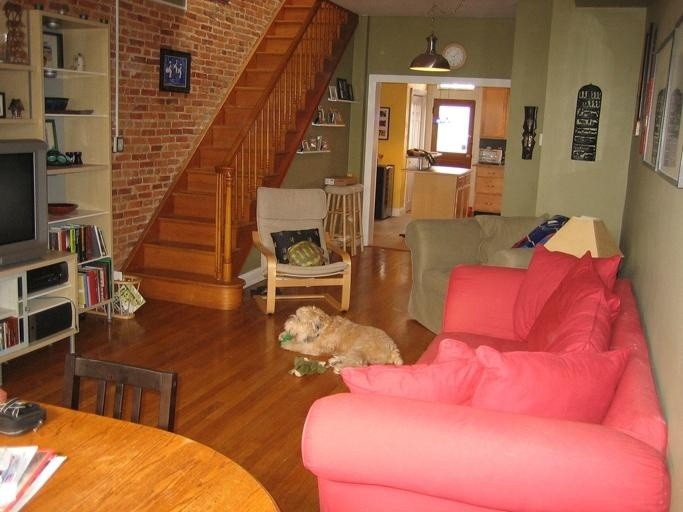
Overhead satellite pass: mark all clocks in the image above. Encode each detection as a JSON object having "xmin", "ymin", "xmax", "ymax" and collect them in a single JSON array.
[{"xmin": 440, "ymin": 42, "xmax": 467, "ymax": 70}]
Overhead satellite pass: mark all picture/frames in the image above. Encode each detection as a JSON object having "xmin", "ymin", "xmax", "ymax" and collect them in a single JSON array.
[
  {"xmin": 41, "ymin": 29, "xmax": 64, "ymax": 69},
  {"xmin": 639, "ymin": 34, "xmax": 676, "ymax": 170},
  {"xmin": 0, "ymin": 91, "xmax": 6, "ymax": 120},
  {"xmin": 376, "ymin": 104, "xmax": 391, "ymax": 142},
  {"xmin": 157, "ymin": 47, "xmax": 192, "ymax": 94},
  {"xmin": 654, "ymin": 16, "xmax": 683, "ymax": 192},
  {"xmin": 44, "ymin": 119, "xmax": 59, "ymax": 155}
]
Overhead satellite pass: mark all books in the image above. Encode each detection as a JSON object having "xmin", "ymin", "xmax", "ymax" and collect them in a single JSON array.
[
  {"xmin": 113, "ymin": 280, "xmax": 146, "ymax": 315},
  {"xmin": 0, "ymin": 316, "xmax": 19, "ymax": 352},
  {"xmin": 49, "ymin": 224, "xmax": 113, "ymax": 308}
]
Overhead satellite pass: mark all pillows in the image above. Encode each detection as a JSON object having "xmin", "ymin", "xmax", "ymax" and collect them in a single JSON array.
[
  {"xmin": 428, "ymin": 335, "xmax": 478, "ymax": 361},
  {"xmin": 465, "ymin": 340, "xmax": 633, "ymax": 422},
  {"xmin": 525, "ymin": 250, "xmax": 626, "ymax": 341},
  {"xmin": 509, "ymin": 244, "xmax": 624, "ymax": 338},
  {"xmin": 269, "ymin": 228, "xmax": 324, "ymax": 266},
  {"xmin": 335, "ymin": 354, "xmax": 478, "ymax": 402},
  {"xmin": 539, "ymin": 287, "xmax": 618, "ymax": 357}
]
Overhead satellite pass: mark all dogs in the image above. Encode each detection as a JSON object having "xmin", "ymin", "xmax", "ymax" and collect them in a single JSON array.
[{"xmin": 277, "ymin": 304, "xmax": 404, "ymax": 376}]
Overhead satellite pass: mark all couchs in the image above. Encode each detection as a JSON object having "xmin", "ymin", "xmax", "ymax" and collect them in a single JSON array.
[
  {"xmin": 403, "ymin": 212, "xmax": 563, "ymax": 336},
  {"xmin": 296, "ymin": 260, "xmax": 671, "ymax": 512}
]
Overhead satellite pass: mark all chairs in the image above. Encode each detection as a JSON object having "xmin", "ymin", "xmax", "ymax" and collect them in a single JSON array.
[
  {"xmin": 248, "ymin": 184, "xmax": 353, "ymax": 318},
  {"xmin": 61, "ymin": 355, "xmax": 179, "ymax": 433}
]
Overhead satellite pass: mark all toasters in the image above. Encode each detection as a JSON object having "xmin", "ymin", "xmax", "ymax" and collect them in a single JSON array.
[{"xmin": 407, "ymin": 155, "xmax": 432, "ymax": 171}]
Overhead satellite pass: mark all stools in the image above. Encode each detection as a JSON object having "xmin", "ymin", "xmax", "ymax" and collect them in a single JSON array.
[
  {"xmin": 348, "ymin": 185, "xmax": 366, "ymax": 256},
  {"xmin": 322, "ymin": 182, "xmax": 356, "ymax": 259}
]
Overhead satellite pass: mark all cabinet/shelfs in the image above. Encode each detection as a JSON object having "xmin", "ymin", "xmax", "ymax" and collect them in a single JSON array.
[
  {"xmin": 406, "ymin": 164, "xmax": 474, "ymax": 219},
  {"xmin": 374, "ymin": 161, "xmax": 395, "ymax": 221},
  {"xmin": 293, "ymin": 96, "xmax": 363, "ymax": 155},
  {"xmin": 472, "ymin": 161, "xmax": 505, "ymax": 215},
  {"xmin": 0, "ymin": 249, "xmax": 81, "ymax": 368},
  {"xmin": 0, "ymin": 0, "xmax": 43, "ymax": 135},
  {"xmin": 28, "ymin": 6, "xmax": 115, "ymax": 328}
]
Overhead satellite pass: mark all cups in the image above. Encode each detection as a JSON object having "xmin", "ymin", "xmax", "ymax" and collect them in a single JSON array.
[{"xmin": 64, "ymin": 151, "xmax": 83, "ymax": 164}]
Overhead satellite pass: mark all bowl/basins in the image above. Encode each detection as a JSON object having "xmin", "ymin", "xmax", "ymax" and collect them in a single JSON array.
[{"xmin": 45, "ymin": 97, "xmax": 68, "ymax": 110}]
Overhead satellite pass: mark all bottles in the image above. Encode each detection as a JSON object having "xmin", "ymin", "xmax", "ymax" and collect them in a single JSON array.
[
  {"xmin": 72, "ymin": 54, "xmax": 83, "ymax": 72},
  {"xmin": 328, "ymin": 112, "xmax": 334, "ymax": 124},
  {"xmin": 318, "ymin": 110, "xmax": 324, "ymax": 124}
]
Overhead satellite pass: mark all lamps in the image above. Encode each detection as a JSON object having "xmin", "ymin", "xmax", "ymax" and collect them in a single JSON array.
[
  {"xmin": 541, "ymin": 214, "xmax": 626, "ymax": 258},
  {"xmin": 519, "ymin": 103, "xmax": 540, "ymax": 162},
  {"xmin": 407, "ymin": 0, "xmax": 453, "ymax": 75}
]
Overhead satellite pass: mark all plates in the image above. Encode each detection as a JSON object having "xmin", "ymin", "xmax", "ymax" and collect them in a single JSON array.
[{"xmin": 47, "ymin": 203, "xmax": 77, "ymax": 214}]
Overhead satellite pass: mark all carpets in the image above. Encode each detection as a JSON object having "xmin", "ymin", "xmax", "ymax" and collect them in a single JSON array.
[{"xmin": 368, "ymin": 211, "xmax": 413, "ymax": 251}]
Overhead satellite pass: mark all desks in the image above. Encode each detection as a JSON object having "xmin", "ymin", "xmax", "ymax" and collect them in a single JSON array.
[{"xmin": 0, "ymin": 390, "xmax": 281, "ymax": 511}]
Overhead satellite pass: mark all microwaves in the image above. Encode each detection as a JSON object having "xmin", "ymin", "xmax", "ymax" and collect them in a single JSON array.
[{"xmin": 479, "ymin": 147, "xmax": 502, "ymax": 164}]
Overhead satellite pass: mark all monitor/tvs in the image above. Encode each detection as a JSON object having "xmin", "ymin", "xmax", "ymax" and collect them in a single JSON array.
[{"xmin": 1, "ymin": 139, "xmax": 49, "ymax": 265}]
